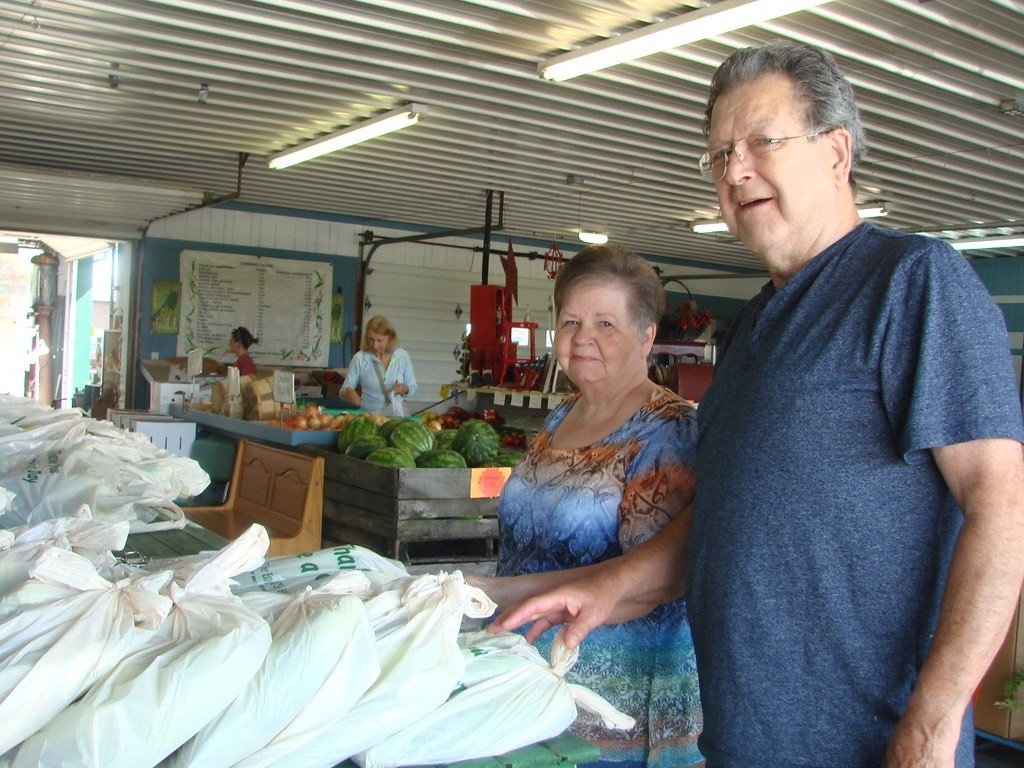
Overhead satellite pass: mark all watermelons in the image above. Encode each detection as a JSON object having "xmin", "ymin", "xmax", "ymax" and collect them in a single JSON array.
[
  {"xmin": 494, "ymin": 451, "xmax": 526, "ymax": 467},
  {"xmin": 337, "ymin": 418, "xmax": 502, "ymax": 468}
]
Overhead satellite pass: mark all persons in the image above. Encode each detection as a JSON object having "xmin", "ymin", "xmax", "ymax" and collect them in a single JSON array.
[
  {"xmin": 229, "ymin": 326, "xmax": 260, "ymax": 376},
  {"xmin": 339, "ymin": 316, "xmax": 418, "ymax": 412},
  {"xmin": 486, "ymin": 45, "xmax": 1023, "ymax": 768},
  {"xmin": 464, "ymin": 245, "xmax": 704, "ymax": 768}
]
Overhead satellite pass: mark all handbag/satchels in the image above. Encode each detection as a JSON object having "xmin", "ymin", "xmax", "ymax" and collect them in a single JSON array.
[
  {"xmin": 378, "ymin": 388, "xmax": 404, "ymax": 416},
  {"xmin": 0, "ymin": 392, "xmax": 638, "ymax": 768}
]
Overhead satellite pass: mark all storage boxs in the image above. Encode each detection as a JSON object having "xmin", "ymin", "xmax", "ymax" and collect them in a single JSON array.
[
  {"xmin": 973, "ymin": 578, "xmax": 1024, "ymax": 740},
  {"xmin": 107, "ymin": 358, "xmax": 199, "ymax": 459}
]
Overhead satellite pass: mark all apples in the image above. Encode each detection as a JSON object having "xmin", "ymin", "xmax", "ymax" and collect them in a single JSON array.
[{"xmin": 430, "ymin": 414, "xmax": 527, "ymax": 450}]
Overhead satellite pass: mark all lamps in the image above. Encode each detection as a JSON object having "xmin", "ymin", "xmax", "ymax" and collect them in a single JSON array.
[
  {"xmin": 945, "ymin": 234, "xmax": 1024, "ymax": 250},
  {"xmin": 537, "ymin": 0, "xmax": 836, "ymax": 85},
  {"xmin": 578, "ymin": 230, "xmax": 609, "ymax": 244},
  {"xmin": 268, "ymin": 103, "xmax": 428, "ymax": 171},
  {"xmin": 689, "ymin": 202, "xmax": 894, "ymax": 234}
]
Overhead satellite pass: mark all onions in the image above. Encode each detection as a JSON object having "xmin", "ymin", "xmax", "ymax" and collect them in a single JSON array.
[{"xmin": 268, "ymin": 404, "xmax": 394, "ymax": 430}]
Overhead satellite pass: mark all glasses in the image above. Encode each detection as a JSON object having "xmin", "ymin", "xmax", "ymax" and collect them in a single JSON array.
[{"xmin": 698, "ymin": 129, "xmax": 834, "ymax": 183}]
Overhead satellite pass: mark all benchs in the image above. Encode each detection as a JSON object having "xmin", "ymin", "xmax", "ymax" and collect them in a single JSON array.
[{"xmin": 176, "ymin": 437, "xmax": 325, "ymax": 560}]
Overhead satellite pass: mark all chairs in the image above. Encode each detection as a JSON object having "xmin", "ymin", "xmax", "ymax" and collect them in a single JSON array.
[{"xmin": 173, "ymin": 439, "xmax": 236, "ymax": 506}]
